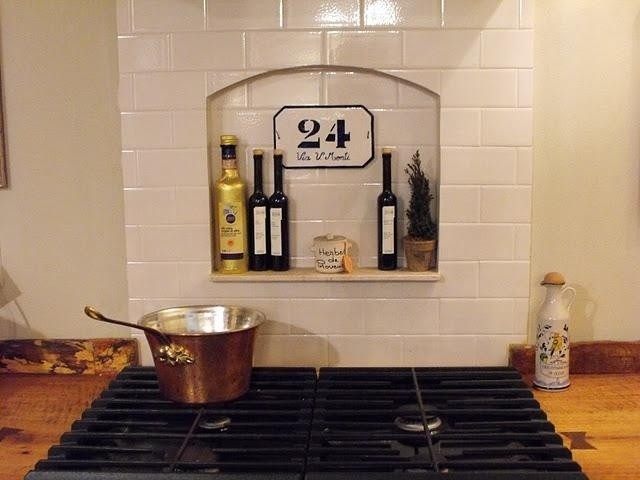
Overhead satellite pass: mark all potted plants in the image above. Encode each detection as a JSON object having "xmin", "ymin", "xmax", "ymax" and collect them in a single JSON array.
[{"xmin": 401, "ymin": 149, "xmax": 436, "ymax": 271}]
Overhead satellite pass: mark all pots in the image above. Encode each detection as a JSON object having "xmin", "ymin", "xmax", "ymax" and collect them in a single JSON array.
[{"xmin": 85, "ymin": 303, "xmax": 267, "ymax": 404}]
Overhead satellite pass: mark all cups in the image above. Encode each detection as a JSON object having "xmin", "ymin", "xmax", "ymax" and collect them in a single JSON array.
[{"xmin": 308, "ymin": 234, "xmax": 347, "ymax": 274}]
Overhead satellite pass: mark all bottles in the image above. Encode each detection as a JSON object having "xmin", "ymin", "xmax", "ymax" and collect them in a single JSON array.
[
  {"xmin": 213, "ymin": 135, "xmax": 249, "ymax": 275},
  {"xmin": 267, "ymin": 148, "xmax": 290, "ymax": 272},
  {"xmin": 375, "ymin": 147, "xmax": 399, "ymax": 273},
  {"xmin": 249, "ymin": 149, "xmax": 268, "ymax": 271},
  {"xmin": 533, "ymin": 273, "xmax": 577, "ymax": 393}
]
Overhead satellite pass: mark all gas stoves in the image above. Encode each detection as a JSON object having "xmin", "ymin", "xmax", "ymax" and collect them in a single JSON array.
[{"xmin": 22, "ymin": 364, "xmax": 590, "ymax": 478}]
